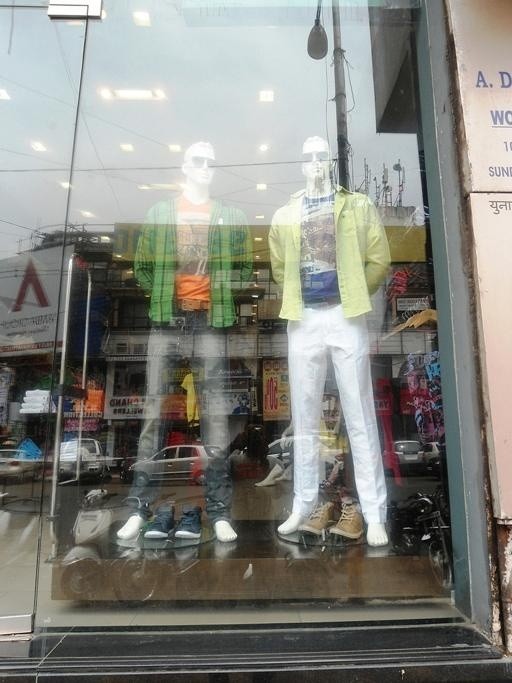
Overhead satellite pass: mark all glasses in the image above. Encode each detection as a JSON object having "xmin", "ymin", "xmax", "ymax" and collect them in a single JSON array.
[
  {"xmin": 300, "ymin": 149, "xmax": 330, "ymax": 162},
  {"xmin": 188, "ymin": 154, "xmax": 218, "ymax": 170}
]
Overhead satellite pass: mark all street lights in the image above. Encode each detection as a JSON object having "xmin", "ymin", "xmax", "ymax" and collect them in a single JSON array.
[{"xmin": 308, "ymin": 0, "xmax": 352, "ymax": 189}]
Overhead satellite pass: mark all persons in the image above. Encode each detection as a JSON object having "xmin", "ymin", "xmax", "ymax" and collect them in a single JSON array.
[
  {"xmin": 116, "ymin": 142, "xmax": 254, "ymax": 544},
  {"xmin": 268, "ymin": 137, "xmax": 391, "ymax": 548},
  {"xmin": 254, "ymin": 424, "xmax": 294, "ymax": 486}
]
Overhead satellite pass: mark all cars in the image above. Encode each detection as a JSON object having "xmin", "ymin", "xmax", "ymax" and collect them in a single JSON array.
[
  {"xmin": 126, "ymin": 443, "xmax": 226, "ymax": 485},
  {"xmin": 264, "ymin": 434, "xmax": 349, "ymax": 474},
  {"xmin": 421, "ymin": 441, "xmax": 444, "ymax": 464},
  {"xmin": 0, "ymin": 447, "xmax": 41, "ymax": 483}
]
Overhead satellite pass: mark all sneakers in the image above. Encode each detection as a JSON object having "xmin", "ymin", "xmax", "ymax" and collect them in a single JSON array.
[
  {"xmin": 296, "ymin": 499, "xmax": 334, "ymax": 533},
  {"xmin": 146, "ymin": 493, "xmax": 175, "ymax": 540},
  {"xmin": 176, "ymin": 501, "xmax": 203, "ymax": 541},
  {"xmin": 330, "ymin": 498, "xmax": 365, "ymax": 541}
]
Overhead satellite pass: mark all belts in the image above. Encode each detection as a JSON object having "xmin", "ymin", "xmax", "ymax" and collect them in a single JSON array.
[{"xmin": 171, "ymin": 296, "xmax": 209, "ymax": 313}]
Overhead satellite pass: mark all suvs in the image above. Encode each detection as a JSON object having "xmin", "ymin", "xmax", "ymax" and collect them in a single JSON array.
[
  {"xmin": 394, "ymin": 439, "xmax": 426, "ymax": 469},
  {"xmin": 60, "ymin": 437, "xmax": 112, "ymax": 480}
]
